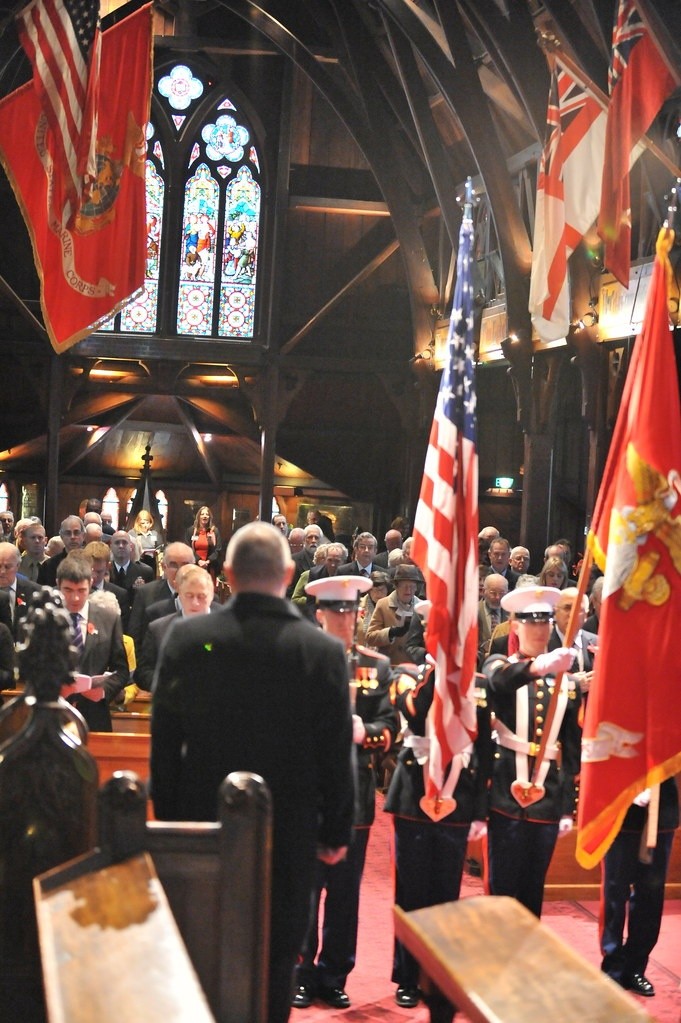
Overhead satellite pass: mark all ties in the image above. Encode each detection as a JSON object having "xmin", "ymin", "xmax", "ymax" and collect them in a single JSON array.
[
  {"xmin": 70, "ymin": 613, "xmax": 85, "ymax": 657},
  {"xmin": 1, "ymin": 587, "xmax": 12, "ymax": 628},
  {"xmin": 31, "ymin": 561, "xmax": 38, "ymax": 582},
  {"xmin": 120, "ymin": 567, "xmax": 125, "ymax": 580},
  {"xmin": 92, "ymin": 586, "xmax": 99, "ymax": 592}
]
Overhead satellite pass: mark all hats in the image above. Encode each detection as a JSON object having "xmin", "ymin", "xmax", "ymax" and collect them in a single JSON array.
[
  {"xmin": 414, "ymin": 600, "xmax": 432, "ymax": 627},
  {"xmin": 389, "ymin": 563, "xmax": 425, "ymax": 584},
  {"xmin": 303, "ymin": 574, "xmax": 373, "ymax": 612},
  {"xmin": 367, "ymin": 571, "xmax": 389, "ymax": 584},
  {"xmin": 500, "ymin": 586, "xmax": 561, "ymax": 623}
]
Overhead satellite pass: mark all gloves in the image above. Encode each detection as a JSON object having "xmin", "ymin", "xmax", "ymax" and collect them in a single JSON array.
[
  {"xmin": 351, "ymin": 714, "xmax": 366, "ymax": 744},
  {"xmin": 556, "ymin": 815, "xmax": 573, "ymax": 837},
  {"xmin": 534, "ymin": 646, "xmax": 578, "ymax": 676},
  {"xmin": 467, "ymin": 821, "xmax": 488, "ymax": 842},
  {"xmin": 633, "ymin": 788, "xmax": 651, "ymax": 807},
  {"xmin": 390, "ymin": 625, "xmax": 407, "ymax": 637}
]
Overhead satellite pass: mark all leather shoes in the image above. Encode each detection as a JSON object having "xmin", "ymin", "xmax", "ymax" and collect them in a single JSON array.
[
  {"xmin": 395, "ymin": 985, "xmax": 419, "ymax": 1007},
  {"xmin": 314, "ymin": 983, "xmax": 350, "ymax": 1008},
  {"xmin": 623, "ymin": 972, "xmax": 654, "ymax": 995},
  {"xmin": 289, "ymin": 980, "xmax": 312, "ymax": 1007}
]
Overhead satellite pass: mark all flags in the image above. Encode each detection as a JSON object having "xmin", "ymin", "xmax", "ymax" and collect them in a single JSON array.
[
  {"xmin": 571, "ymin": 226, "xmax": 681, "ymax": 866},
  {"xmin": 597, "ymin": 0, "xmax": 681, "ymax": 288},
  {"xmin": 411, "ymin": 181, "xmax": 482, "ymax": 822},
  {"xmin": 0, "ymin": 3, "xmax": 156, "ymax": 355},
  {"xmin": 15, "ymin": 0, "xmax": 103, "ymax": 230},
  {"xmin": 526, "ymin": 47, "xmax": 648, "ymax": 342}
]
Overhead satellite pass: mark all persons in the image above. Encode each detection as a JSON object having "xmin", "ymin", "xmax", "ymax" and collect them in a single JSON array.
[
  {"xmin": 1, "ymin": 482, "xmax": 678, "ymax": 1023},
  {"xmin": 148, "ymin": 519, "xmax": 356, "ymax": 1023}
]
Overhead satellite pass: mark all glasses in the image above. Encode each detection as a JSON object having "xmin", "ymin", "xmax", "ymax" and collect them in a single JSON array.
[
  {"xmin": 484, "ymin": 587, "xmax": 508, "ymax": 597},
  {"xmin": 289, "ymin": 541, "xmax": 305, "ymax": 546}
]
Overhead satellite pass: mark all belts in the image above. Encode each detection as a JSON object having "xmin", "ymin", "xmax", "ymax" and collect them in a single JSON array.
[
  {"xmin": 403, "ymin": 736, "xmax": 474, "ymax": 753},
  {"xmin": 496, "ymin": 733, "xmax": 559, "ymax": 760}
]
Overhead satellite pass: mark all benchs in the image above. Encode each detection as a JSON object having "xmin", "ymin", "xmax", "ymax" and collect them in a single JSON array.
[{"xmin": 0, "ymin": 680, "xmax": 156, "ymax": 823}]
